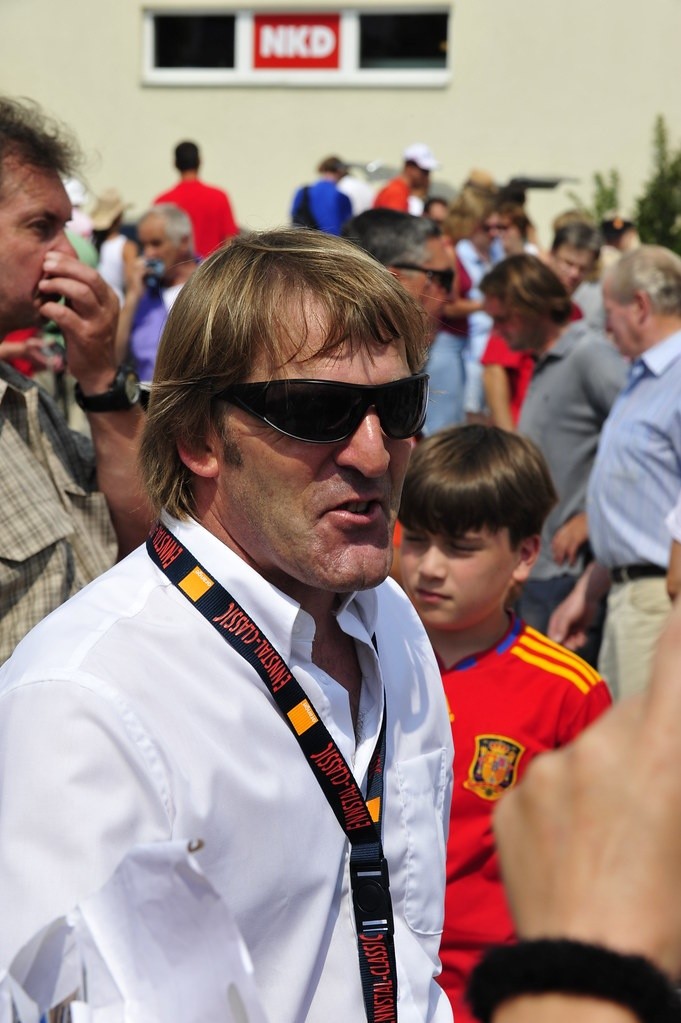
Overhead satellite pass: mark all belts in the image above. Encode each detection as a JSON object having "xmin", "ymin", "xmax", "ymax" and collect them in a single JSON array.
[{"xmin": 612, "ymin": 562, "xmax": 664, "ymax": 582}]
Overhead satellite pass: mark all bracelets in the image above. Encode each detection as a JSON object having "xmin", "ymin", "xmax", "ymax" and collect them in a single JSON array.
[{"xmin": 466, "ymin": 936, "xmax": 681, "ymax": 1022}]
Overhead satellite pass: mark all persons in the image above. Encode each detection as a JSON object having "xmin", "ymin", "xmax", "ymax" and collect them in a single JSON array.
[
  {"xmin": 154, "ymin": 142, "xmax": 241, "ymax": 260},
  {"xmin": 1, "ymin": 230, "xmax": 453, "ymax": 1023},
  {"xmin": 0, "ymin": 95, "xmax": 161, "ymax": 666},
  {"xmin": 291, "ymin": 146, "xmax": 439, "ymax": 238},
  {"xmin": 61, "ymin": 177, "xmax": 199, "ymax": 413},
  {"xmin": 397, "ymin": 424, "xmax": 611, "ymax": 1023},
  {"xmin": 464, "ymin": 587, "xmax": 681, "ymax": 1023},
  {"xmin": 424, "ymin": 169, "xmax": 538, "ymax": 249},
  {"xmin": 346, "ymin": 207, "xmax": 681, "ymax": 703}
]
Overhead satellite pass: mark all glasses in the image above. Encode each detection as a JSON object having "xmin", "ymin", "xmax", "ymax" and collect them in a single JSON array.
[
  {"xmin": 481, "ymin": 222, "xmax": 513, "ymax": 233},
  {"xmin": 390, "ymin": 262, "xmax": 456, "ymax": 291},
  {"xmin": 214, "ymin": 370, "xmax": 431, "ymax": 442}
]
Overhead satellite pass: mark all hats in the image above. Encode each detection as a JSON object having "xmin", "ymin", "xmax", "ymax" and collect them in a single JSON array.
[
  {"xmin": 599, "ymin": 218, "xmax": 634, "ymax": 243},
  {"xmin": 403, "ymin": 142, "xmax": 438, "ymax": 169}
]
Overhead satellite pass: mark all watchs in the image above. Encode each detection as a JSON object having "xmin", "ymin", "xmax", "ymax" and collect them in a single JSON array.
[{"xmin": 74, "ymin": 367, "xmax": 141, "ymax": 412}]
